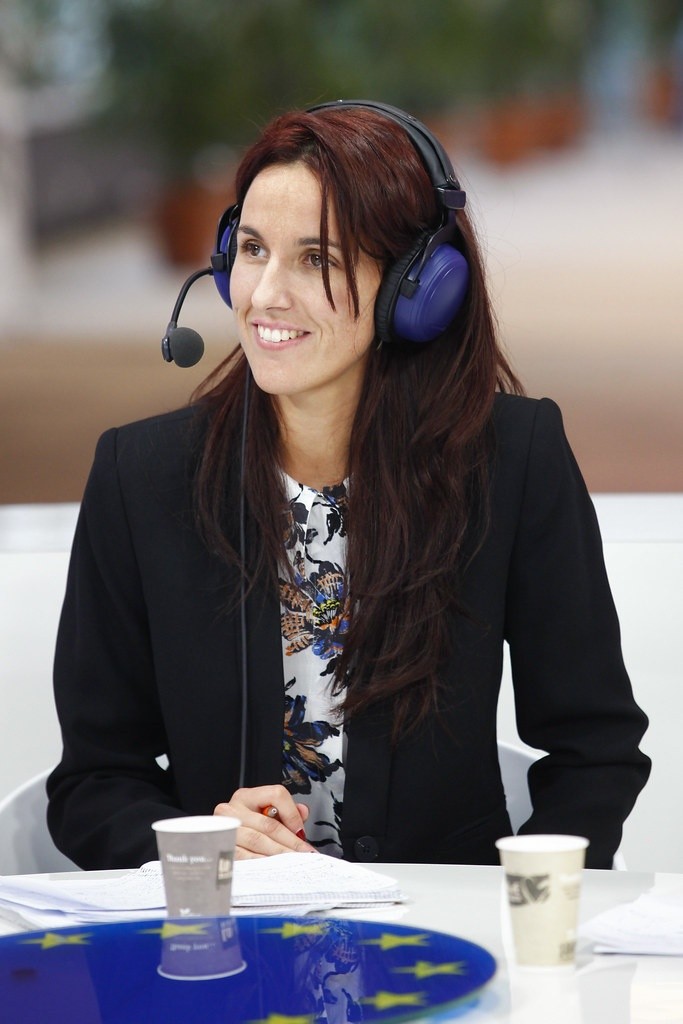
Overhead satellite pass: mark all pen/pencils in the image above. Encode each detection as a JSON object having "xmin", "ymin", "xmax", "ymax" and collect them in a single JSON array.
[{"xmin": 262, "ymin": 805, "xmax": 278, "ymax": 818}]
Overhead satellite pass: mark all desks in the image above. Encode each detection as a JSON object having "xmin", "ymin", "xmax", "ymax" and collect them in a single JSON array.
[{"xmin": 0, "ymin": 858, "xmax": 683, "ymax": 1024}]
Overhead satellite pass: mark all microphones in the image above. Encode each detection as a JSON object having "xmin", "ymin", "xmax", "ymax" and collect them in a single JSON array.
[{"xmin": 162, "ymin": 266, "xmax": 213, "ymax": 369}]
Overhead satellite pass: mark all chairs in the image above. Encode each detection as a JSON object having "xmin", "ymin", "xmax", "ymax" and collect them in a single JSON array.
[{"xmin": 0, "ymin": 744, "xmax": 636, "ymax": 876}]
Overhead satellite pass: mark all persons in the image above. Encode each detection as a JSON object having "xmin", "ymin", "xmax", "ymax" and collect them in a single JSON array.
[{"xmin": 46, "ymin": 100, "xmax": 652, "ymax": 870}]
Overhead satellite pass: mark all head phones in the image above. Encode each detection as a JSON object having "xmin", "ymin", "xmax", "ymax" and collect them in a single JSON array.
[{"xmin": 209, "ymin": 97, "xmax": 471, "ymax": 346}]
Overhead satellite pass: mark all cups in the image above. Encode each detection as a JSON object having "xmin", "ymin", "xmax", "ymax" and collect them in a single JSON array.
[
  {"xmin": 151, "ymin": 816, "xmax": 241, "ymax": 918},
  {"xmin": 157, "ymin": 918, "xmax": 247, "ymax": 981},
  {"xmin": 495, "ymin": 833, "xmax": 588, "ymax": 966}
]
